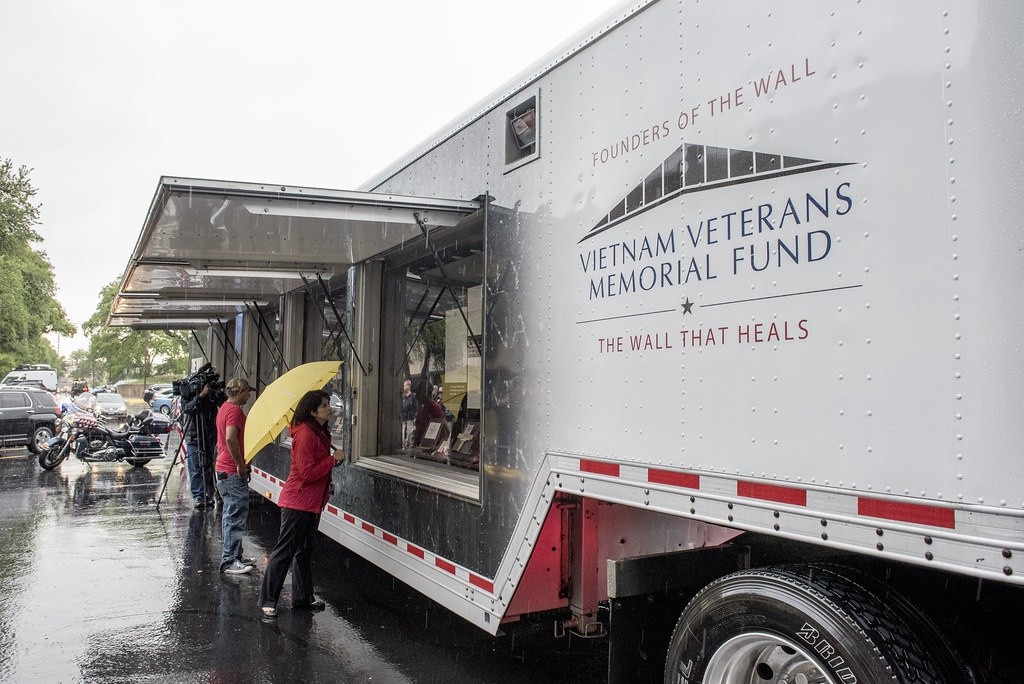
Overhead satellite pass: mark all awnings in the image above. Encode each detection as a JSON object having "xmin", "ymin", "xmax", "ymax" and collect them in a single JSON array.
[{"xmin": 104, "ymin": 174, "xmax": 480, "ymax": 332}]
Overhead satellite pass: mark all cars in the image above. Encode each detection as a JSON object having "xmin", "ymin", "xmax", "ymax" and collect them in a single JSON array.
[
  {"xmin": 153, "ymin": 388, "xmax": 175, "ymax": 415},
  {"xmin": 91, "ymin": 386, "xmax": 118, "ymax": 395},
  {"xmin": 94, "ymin": 393, "xmax": 128, "ymax": 422}
]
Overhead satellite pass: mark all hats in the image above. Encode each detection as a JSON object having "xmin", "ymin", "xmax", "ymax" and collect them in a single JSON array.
[{"xmin": 226, "ymin": 377, "xmax": 257, "ymax": 392}]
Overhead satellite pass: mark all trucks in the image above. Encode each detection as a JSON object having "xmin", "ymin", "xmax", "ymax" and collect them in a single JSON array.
[{"xmin": 104, "ymin": 0, "xmax": 1024, "ymax": 684}]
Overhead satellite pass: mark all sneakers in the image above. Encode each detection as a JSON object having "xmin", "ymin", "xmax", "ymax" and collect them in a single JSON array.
[
  {"xmin": 224, "ymin": 560, "xmax": 252, "ymax": 573},
  {"xmin": 309, "ymin": 601, "xmax": 325, "ymax": 609},
  {"xmin": 241, "ymin": 556, "xmax": 257, "ymax": 565},
  {"xmin": 259, "ymin": 606, "xmax": 277, "ymax": 623},
  {"xmin": 206, "ymin": 494, "xmax": 215, "ymax": 504},
  {"xmin": 193, "ymin": 496, "xmax": 203, "ymax": 507}
]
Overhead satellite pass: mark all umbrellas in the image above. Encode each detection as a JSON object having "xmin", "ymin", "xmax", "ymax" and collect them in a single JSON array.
[{"xmin": 243, "ymin": 361, "xmax": 345, "ymax": 467}]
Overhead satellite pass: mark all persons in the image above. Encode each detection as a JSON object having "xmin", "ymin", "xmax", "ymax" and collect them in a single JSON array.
[
  {"xmin": 256, "ymin": 390, "xmax": 346, "ymax": 616},
  {"xmin": 411, "ymin": 380, "xmax": 450, "ymax": 465},
  {"xmin": 181, "ymin": 363, "xmax": 229, "ymax": 508},
  {"xmin": 215, "ymin": 378, "xmax": 257, "ymax": 574},
  {"xmin": 402, "ymin": 380, "xmax": 414, "ymax": 421}
]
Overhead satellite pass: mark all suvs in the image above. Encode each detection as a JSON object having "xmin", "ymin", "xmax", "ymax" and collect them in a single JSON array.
[
  {"xmin": 143, "ymin": 384, "xmax": 178, "ymax": 408},
  {"xmin": 71, "ymin": 379, "xmax": 88, "ymax": 397},
  {"xmin": 0, "ymin": 387, "xmax": 62, "ymax": 454},
  {"xmin": 0, "ymin": 380, "xmax": 48, "ymax": 392}
]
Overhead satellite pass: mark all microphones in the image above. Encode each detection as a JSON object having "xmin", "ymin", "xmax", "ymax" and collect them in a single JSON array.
[{"xmin": 189, "ymin": 363, "xmax": 212, "ymax": 381}]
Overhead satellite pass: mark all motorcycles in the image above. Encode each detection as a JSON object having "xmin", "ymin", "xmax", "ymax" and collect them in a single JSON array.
[{"xmin": 38, "ymin": 392, "xmax": 171, "ymax": 470}]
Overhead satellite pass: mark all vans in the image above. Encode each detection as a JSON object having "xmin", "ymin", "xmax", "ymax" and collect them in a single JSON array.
[{"xmin": 0, "ymin": 364, "xmax": 58, "ymax": 395}]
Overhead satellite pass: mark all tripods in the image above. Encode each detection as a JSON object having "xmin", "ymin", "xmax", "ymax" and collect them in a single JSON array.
[{"xmin": 155, "ymin": 394, "xmax": 218, "ymax": 516}]
